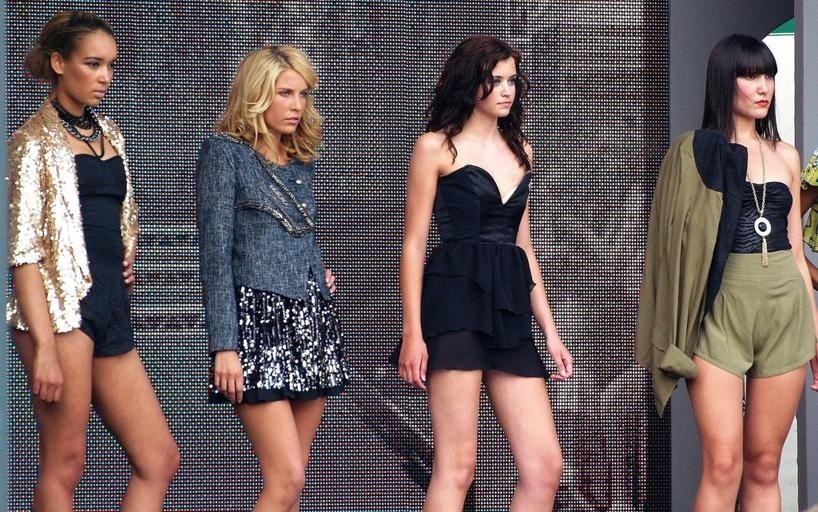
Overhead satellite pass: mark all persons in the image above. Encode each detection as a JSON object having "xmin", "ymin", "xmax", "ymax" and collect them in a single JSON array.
[
  {"xmin": 635, "ymin": 32, "xmax": 818, "ymax": 512},
  {"xmin": 7, "ymin": 12, "xmax": 181, "ymax": 511},
  {"xmin": 193, "ymin": 45, "xmax": 350, "ymax": 512},
  {"xmin": 386, "ymin": 35, "xmax": 575, "ymax": 511}
]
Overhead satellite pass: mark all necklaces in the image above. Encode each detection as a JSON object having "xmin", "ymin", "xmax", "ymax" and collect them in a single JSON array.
[
  {"xmin": 745, "ymin": 128, "xmax": 773, "ymax": 268},
  {"xmin": 50, "ymin": 97, "xmax": 106, "ymax": 159}
]
